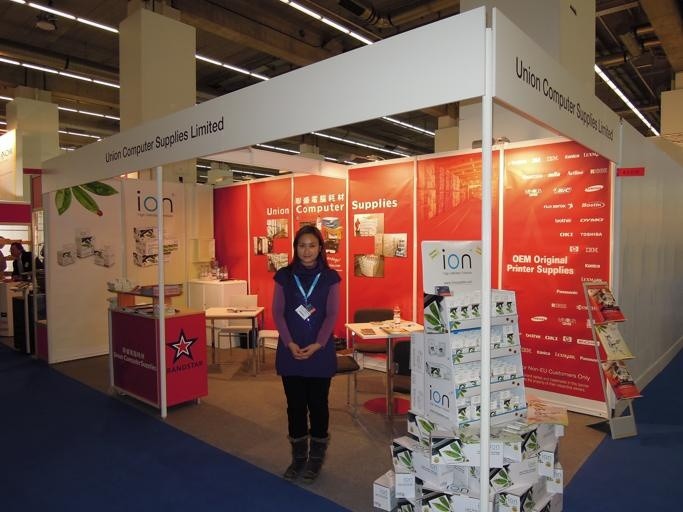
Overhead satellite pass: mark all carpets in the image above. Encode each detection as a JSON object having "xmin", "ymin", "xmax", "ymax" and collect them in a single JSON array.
[{"xmin": 52, "ymin": 341, "xmax": 609, "ymax": 510}]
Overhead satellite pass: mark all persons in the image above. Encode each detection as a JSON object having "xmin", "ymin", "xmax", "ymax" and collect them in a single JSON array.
[
  {"xmin": 0, "ymin": 242, "xmax": 43, "ymax": 289},
  {"xmin": 271, "ymin": 225, "xmax": 343, "ymax": 484}
]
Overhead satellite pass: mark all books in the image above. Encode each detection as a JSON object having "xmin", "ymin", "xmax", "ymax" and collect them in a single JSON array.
[
  {"xmin": 525, "ymin": 398, "xmax": 570, "ymax": 427},
  {"xmin": 586, "ymin": 288, "xmax": 626, "ymax": 324},
  {"xmin": 600, "ymin": 359, "xmax": 640, "ymax": 399},
  {"xmin": 380, "ymin": 326, "xmax": 409, "ymax": 335},
  {"xmin": 593, "ymin": 322, "xmax": 635, "ymax": 361},
  {"xmin": 360, "ymin": 328, "xmax": 375, "ymax": 335},
  {"xmin": 141, "ymin": 285, "xmax": 183, "ymax": 296}
]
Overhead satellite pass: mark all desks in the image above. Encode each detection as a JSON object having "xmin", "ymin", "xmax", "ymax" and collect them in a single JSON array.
[{"xmin": 202, "ymin": 307, "xmax": 265, "ymax": 377}]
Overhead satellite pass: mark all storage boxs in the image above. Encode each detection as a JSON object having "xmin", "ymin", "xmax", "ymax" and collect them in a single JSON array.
[{"xmin": 369, "ymin": 409, "xmax": 566, "ymax": 512}]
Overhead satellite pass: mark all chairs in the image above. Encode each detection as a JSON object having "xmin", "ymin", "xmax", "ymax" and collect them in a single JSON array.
[
  {"xmin": 218, "ymin": 295, "xmax": 259, "ymax": 365},
  {"xmin": 331, "ymin": 309, "xmax": 413, "ymax": 418},
  {"xmin": 257, "ymin": 328, "xmax": 280, "ymax": 374}
]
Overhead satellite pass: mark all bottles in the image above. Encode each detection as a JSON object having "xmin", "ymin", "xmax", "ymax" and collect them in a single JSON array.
[
  {"xmin": 217, "ymin": 265, "xmax": 228, "ymax": 280},
  {"xmin": 199, "ymin": 265, "xmax": 209, "ymax": 277},
  {"xmin": 393, "ymin": 304, "xmax": 401, "ymax": 324}
]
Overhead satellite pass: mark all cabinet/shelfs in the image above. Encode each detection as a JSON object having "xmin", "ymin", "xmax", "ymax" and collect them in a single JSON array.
[
  {"xmin": 188, "ymin": 279, "xmax": 248, "ymax": 349},
  {"xmin": 423, "ymin": 289, "xmax": 531, "ymax": 435}
]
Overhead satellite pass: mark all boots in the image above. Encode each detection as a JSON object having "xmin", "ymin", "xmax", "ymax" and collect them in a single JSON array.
[
  {"xmin": 301, "ymin": 433, "xmax": 331, "ymax": 484},
  {"xmin": 282, "ymin": 432, "xmax": 309, "ymax": 481}
]
